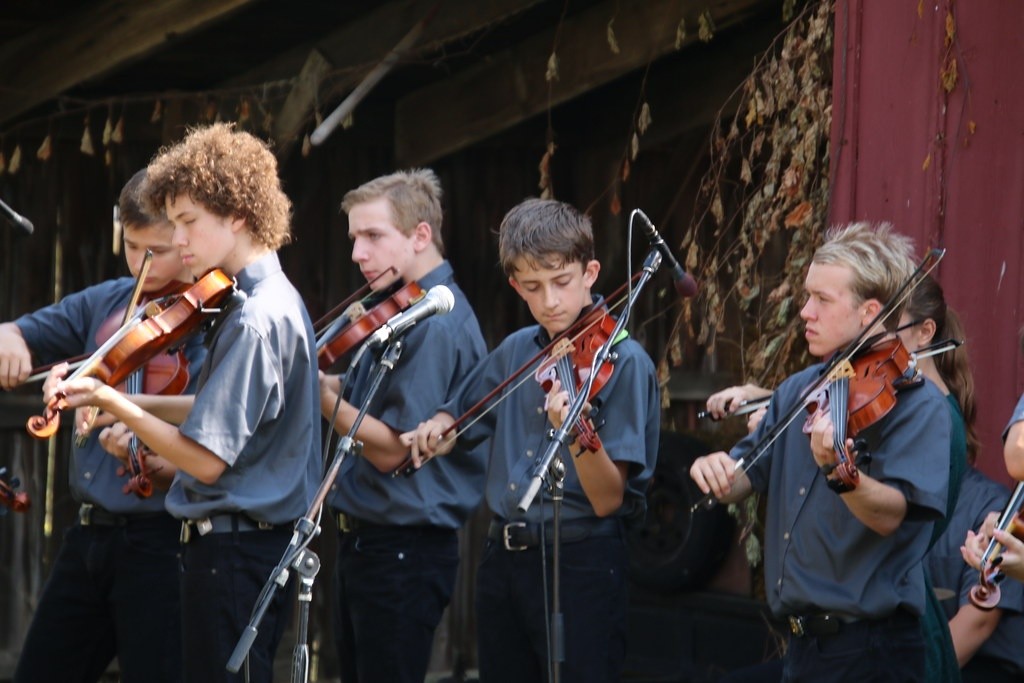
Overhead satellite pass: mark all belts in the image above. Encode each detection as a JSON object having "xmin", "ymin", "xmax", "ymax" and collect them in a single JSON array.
[
  {"xmin": 785, "ymin": 614, "xmax": 842, "ymax": 637},
  {"xmin": 335, "ymin": 511, "xmax": 376, "ymax": 534},
  {"xmin": 78, "ymin": 502, "xmax": 172, "ymax": 531},
  {"xmin": 179, "ymin": 513, "xmax": 293, "ymax": 544},
  {"xmin": 487, "ymin": 516, "xmax": 621, "ymax": 552}
]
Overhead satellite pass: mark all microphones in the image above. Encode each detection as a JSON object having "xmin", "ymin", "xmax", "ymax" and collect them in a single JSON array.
[
  {"xmin": 367, "ymin": 285, "xmax": 455, "ymax": 346},
  {"xmin": 635, "ymin": 208, "xmax": 698, "ymax": 297},
  {"xmin": 0, "ymin": 199, "xmax": 34, "ymax": 236}
]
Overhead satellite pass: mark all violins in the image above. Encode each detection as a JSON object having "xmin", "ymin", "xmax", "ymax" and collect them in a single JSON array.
[
  {"xmin": 534, "ymin": 303, "xmax": 618, "ymax": 460},
  {"xmin": 91, "ymin": 278, "xmax": 196, "ymax": 502},
  {"xmin": 966, "ymin": 480, "xmax": 1024, "ymax": 615},
  {"xmin": 24, "ymin": 267, "xmax": 238, "ymax": 441},
  {"xmin": 316, "ymin": 278, "xmax": 425, "ymax": 372},
  {"xmin": 800, "ymin": 338, "xmax": 912, "ymax": 491},
  {"xmin": 0, "ymin": 466, "xmax": 31, "ymax": 515}
]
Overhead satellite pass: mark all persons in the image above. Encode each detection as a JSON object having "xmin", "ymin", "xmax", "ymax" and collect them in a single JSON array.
[
  {"xmin": 924, "ymin": 394, "xmax": 1023, "ymax": 683},
  {"xmin": 315, "ymin": 168, "xmax": 489, "ymax": 681},
  {"xmin": 399, "ymin": 197, "xmax": 660, "ymax": 683},
  {"xmin": 690, "ymin": 222, "xmax": 979, "ymax": 683},
  {"xmin": 0, "ymin": 125, "xmax": 324, "ymax": 683}
]
